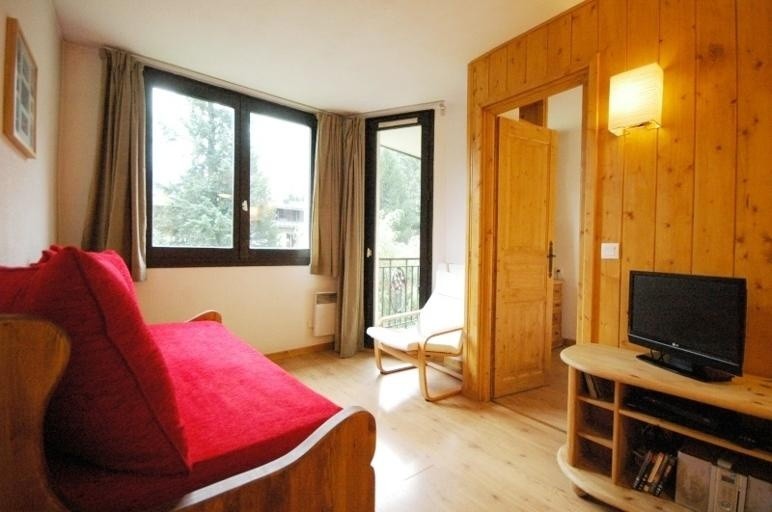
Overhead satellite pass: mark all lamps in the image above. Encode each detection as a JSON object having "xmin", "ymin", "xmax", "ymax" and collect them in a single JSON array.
[{"xmin": 603, "ymin": 60, "xmax": 665, "ymax": 137}]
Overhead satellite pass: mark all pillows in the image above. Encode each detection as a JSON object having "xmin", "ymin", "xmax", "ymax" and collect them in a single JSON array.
[{"xmin": 0, "ymin": 245, "xmax": 193, "ymax": 479}]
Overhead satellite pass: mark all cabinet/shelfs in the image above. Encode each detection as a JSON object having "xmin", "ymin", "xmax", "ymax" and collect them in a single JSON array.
[
  {"xmin": 553, "ymin": 342, "xmax": 772, "ymax": 512},
  {"xmin": 550, "ymin": 279, "xmax": 563, "ymax": 349}
]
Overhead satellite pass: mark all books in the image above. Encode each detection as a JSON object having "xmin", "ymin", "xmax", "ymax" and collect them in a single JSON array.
[
  {"xmin": 643, "ymin": 452, "xmax": 664, "ymax": 491},
  {"xmin": 649, "ymin": 453, "xmax": 672, "ymax": 495},
  {"xmin": 656, "ymin": 454, "xmax": 678, "ymax": 497},
  {"xmin": 632, "ymin": 447, "xmax": 653, "ymax": 489},
  {"xmin": 637, "ymin": 451, "xmax": 660, "ymax": 491}
]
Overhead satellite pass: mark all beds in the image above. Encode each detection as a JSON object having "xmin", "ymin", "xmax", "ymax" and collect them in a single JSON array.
[{"xmin": 1, "ymin": 243, "xmax": 376, "ymax": 512}]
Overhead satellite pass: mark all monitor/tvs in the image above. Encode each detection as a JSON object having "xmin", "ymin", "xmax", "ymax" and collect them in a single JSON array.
[{"xmin": 627, "ymin": 271, "xmax": 748, "ymax": 384}]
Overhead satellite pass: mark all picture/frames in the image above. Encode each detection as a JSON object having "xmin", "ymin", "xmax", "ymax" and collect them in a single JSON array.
[{"xmin": 4, "ymin": 16, "xmax": 42, "ymax": 160}]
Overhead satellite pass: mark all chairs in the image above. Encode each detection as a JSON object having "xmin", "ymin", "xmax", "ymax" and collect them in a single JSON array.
[{"xmin": 367, "ymin": 270, "xmax": 466, "ymax": 402}]
